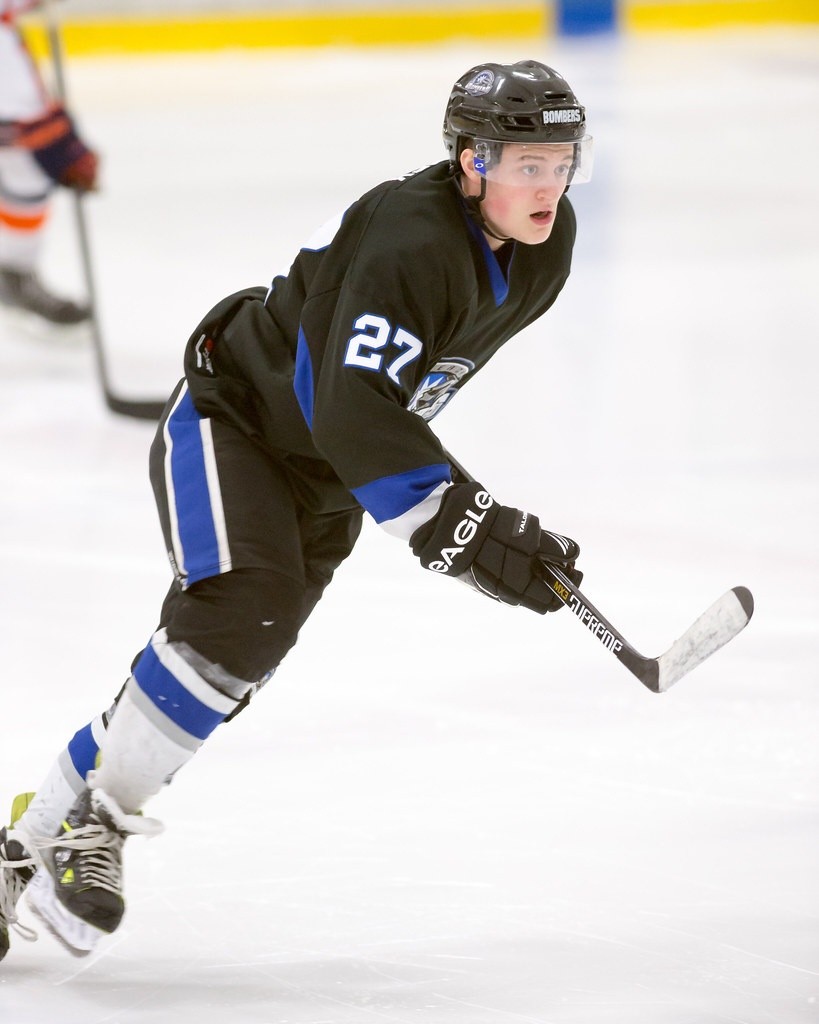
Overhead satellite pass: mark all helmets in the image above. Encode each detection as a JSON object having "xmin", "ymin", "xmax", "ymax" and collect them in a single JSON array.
[{"xmin": 442, "ymin": 59, "xmax": 587, "ymax": 243}]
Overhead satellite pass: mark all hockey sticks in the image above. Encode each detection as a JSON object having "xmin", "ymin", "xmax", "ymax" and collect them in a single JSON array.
[
  {"xmin": 422, "ymin": 417, "xmax": 757, "ymax": 696},
  {"xmin": 45, "ymin": 20, "xmax": 175, "ymax": 422}
]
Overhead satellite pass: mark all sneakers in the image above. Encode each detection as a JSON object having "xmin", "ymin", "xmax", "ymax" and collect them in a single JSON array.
[
  {"xmin": 0, "ymin": 825, "xmax": 41, "ymax": 963},
  {"xmin": 25, "ymin": 787, "xmax": 164, "ymax": 953},
  {"xmin": 0, "ymin": 265, "xmax": 92, "ymax": 324}
]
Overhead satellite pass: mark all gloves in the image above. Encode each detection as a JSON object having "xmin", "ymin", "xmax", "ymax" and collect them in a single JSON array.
[
  {"xmin": 409, "ymin": 481, "xmax": 583, "ymax": 615},
  {"xmin": 11, "ymin": 101, "xmax": 98, "ymax": 196}
]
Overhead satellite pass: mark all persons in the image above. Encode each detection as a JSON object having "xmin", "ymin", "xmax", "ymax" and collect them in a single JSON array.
[
  {"xmin": 1, "ymin": 58, "xmax": 583, "ymax": 953},
  {"xmin": 1, "ymin": 1, "xmax": 99, "ymax": 327}
]
